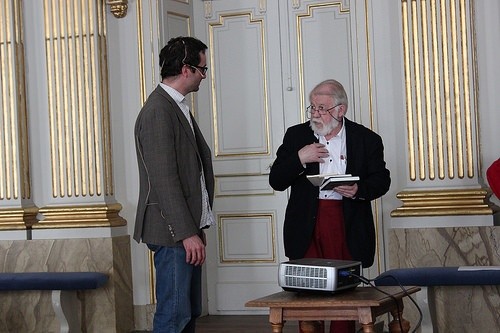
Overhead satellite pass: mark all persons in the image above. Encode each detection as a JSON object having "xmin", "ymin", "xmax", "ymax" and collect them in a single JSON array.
[
  {"xmin": 268, "ymin": 80, "xmax": 391, "ymax": 333},
  {"xmin": 133, "ymin": 36, "xmax": 216, "ymax": 333}
]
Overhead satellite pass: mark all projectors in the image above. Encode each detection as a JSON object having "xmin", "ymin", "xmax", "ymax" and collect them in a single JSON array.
[{"xmin": 276, "ymin": 258, "xmax": 362, "ymax": 297}]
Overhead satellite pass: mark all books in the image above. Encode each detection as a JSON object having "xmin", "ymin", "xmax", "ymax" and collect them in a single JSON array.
[{"xmin": 307, "ymin": 174, "xmax": 360, "ymax": 190}]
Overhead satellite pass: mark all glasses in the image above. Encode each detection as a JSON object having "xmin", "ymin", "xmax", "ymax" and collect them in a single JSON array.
[
  {"xmin": 182, "ymin": 63, "xmax": 208, "ymax": 75},
  {"xmin": 307, "ymin": 103, "xmax": 342, "ymax": 115}
]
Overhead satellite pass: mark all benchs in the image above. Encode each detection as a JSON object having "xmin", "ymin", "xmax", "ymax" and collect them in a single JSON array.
[
  {"xmin": 375, "ymin": 265, "xmax": 500, "ymax": 333},
  {"xmin": 0, "ymin": 272, "xmax": 107, "ymax": 333}
]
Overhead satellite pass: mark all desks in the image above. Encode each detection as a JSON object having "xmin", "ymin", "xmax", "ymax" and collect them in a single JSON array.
[{"xmin": 244, "ymin": 285, "xmax": 421, "ymax": 333}]
